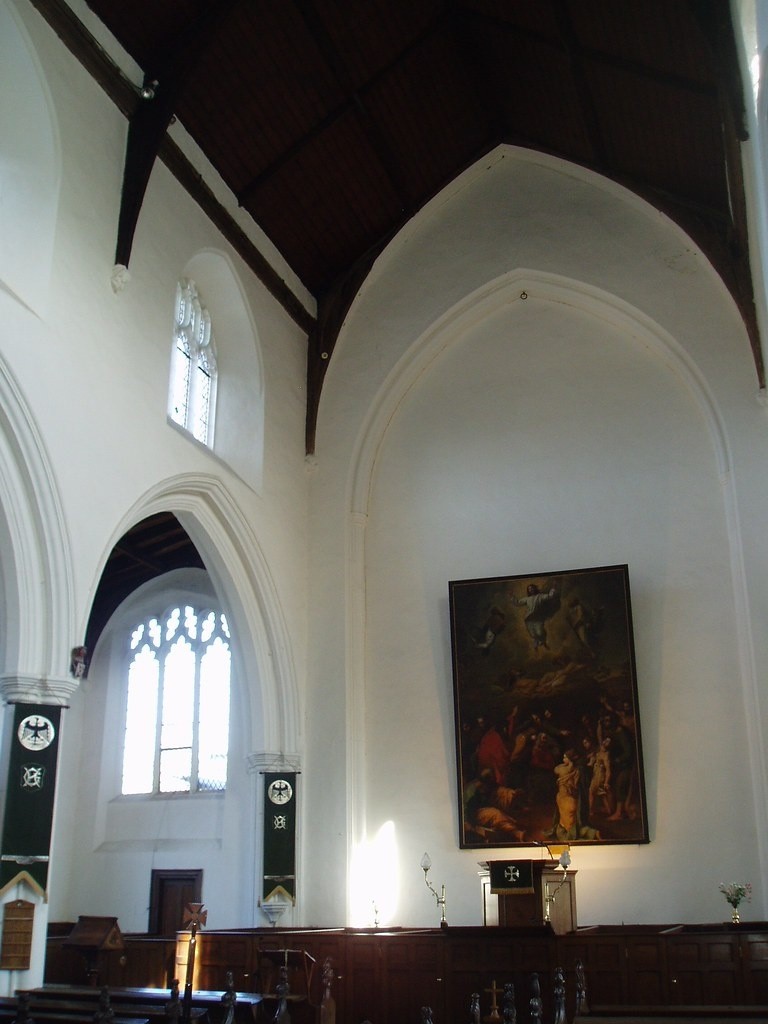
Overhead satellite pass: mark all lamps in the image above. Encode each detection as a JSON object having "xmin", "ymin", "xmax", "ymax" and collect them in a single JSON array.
[
  {"xmin": 421, "ymin": 851, "xmax": 449, "ymax": 927},
  {"xmin": 544, "ymin": 851, "xmax": 570, "ymax": 936}
]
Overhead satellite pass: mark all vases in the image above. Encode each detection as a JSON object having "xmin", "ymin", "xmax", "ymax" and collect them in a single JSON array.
[{"xmin": 732, "ymin": 908, "xmax": 739, "ymax": 923}]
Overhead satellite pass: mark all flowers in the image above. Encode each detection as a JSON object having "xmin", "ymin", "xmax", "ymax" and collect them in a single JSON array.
[{"xmin": 719, "ymin": 881, "xmax": 753, "ymax": 908}]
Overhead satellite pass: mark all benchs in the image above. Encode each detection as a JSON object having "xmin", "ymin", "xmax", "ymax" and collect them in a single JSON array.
[{"xmin": 0, "ymin": 985, "xmax": 309, "ymax": 1024}]
